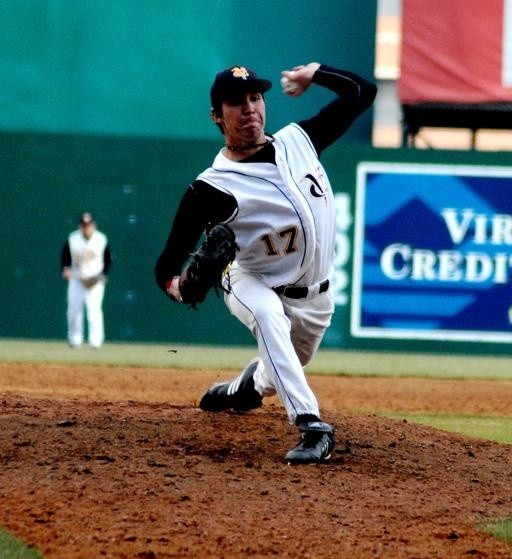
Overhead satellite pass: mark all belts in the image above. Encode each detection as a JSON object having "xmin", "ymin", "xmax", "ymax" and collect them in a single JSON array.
[{"xmin": 273, "ymin": 278, "xmax": 329, "ymax": 299}]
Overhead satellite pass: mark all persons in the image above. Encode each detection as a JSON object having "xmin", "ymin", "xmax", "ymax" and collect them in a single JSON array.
[
  {"xmin": 60, "ymin": 210, "xmax": 113, "ymax": 352},
  {"xmin": 153, "ymin": 60, "xmax": 379, "ymax": 464}
]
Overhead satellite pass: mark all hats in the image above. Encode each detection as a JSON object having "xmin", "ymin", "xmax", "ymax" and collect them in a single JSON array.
[
  {"xmin": 210, "ymin": 65, "xmax": 273, "ymax": 108},
  {"xmin": 80, "ymin": 211, "xmax": 94, "ymax": 226}
]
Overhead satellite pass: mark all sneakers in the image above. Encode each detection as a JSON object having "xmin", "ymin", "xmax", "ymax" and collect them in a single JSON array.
[
  {"xmin": 284, "ymin": 421, "xmax": 337, "ymax": 465},
  {"xmin": 198, "ymin": 356, "xmax": 262, "ymax": 415}
]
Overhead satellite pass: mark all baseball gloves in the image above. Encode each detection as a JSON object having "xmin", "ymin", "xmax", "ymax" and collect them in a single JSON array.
[
  {"xmin": 177, "ymin": 224, "xmax": 236, "ymax": 306},
  {"xmin": 80, "ymin": 276, "xmax": 97, "ymax": 290}
]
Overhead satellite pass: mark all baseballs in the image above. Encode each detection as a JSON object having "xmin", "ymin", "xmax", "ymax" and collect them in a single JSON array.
[{"xmin": 280, "ymin": 71, "xmax": 298, "ymax": 90}]
